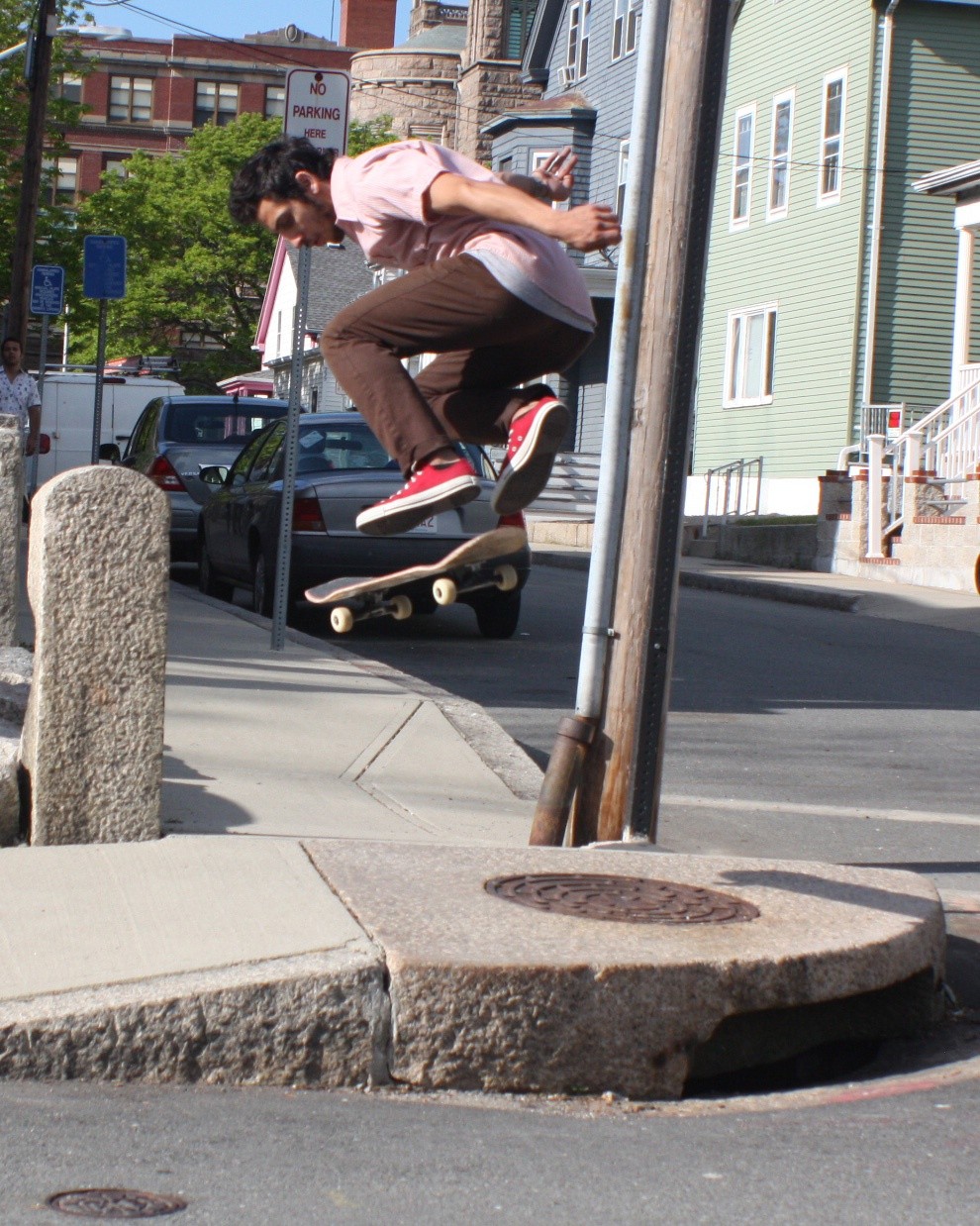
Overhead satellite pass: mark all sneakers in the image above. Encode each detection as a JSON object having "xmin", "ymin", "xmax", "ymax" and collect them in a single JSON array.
[
  {"xmin": 492, "ymin": 395, "xmax": 570, "ymax": 516},
  {"xmin": 358, "ymin": 461, "xmax": 479, "ymax": 537}
]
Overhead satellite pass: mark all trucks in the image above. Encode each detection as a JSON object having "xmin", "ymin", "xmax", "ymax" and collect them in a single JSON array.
[{"xmin": 22, "ymin": 371, "xmax": 185, "ymax": 521}]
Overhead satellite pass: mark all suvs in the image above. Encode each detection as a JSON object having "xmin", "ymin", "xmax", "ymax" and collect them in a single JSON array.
[{"xmin": 98, "ymin": 394, "xmax": 307, "ymax": 570}]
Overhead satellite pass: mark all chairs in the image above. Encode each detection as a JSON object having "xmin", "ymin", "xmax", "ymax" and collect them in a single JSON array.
[{"xmin": 300, "ymin": 457, "xmax": 331, "ymax": 471}]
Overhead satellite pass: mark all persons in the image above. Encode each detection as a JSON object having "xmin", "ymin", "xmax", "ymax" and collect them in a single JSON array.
[
  {"xmin": 225, "ymin": 137, "xmax": 623, "ymax": 537},
  {"xmin": 0, "ymin": 338, "xmax": 43, "ymax": 456}
]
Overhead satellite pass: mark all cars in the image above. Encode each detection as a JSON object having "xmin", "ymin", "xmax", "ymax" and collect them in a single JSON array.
[{"xmin": 197, "ymin": 412, "xmax": 534, "ymax": 639}]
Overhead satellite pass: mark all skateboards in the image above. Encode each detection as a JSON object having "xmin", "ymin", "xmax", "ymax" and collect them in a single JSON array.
[{"xmin": 303, "ymin": 523, "xmax": 529, "ymax": 634}]
